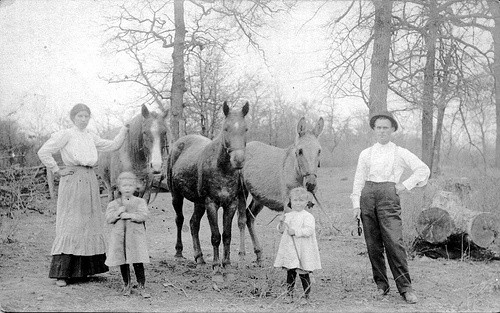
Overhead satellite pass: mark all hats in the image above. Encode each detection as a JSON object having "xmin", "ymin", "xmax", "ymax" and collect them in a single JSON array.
[
  {"xmin": 370, "ymin": 110, "xmax": 398, "ymax": 132},
  {"xmin": 70, "ymin": 103, "xmax": 90, "ymax": 120}
]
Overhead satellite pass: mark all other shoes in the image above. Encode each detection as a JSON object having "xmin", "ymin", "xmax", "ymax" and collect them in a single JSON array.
[
  {"xmin": 138, "ymin": 283, "xmax": 151, "ymax": 298},
  {"xmin": 400, "ymin": 291, "xmax": 418, "ymax": 304},
  {"xmin": 373, "ymin": 288, "xmax": 390, "ymax": 298},
  {"xmin": 124, "ymin": 286, "xmax": 132, "ymax": 296},
  {"xmin": 301, "ymin": 297, "xmax": 308, "ymax": 305},
  {"xmin": 287, "ymin": 295, "xmax": 293, "ymax": 304},
  {"xmin": 55, "ymin": 280, "xmax": 66, "ymax": 287}
]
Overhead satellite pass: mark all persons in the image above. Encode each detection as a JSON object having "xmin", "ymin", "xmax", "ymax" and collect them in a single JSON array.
[
  {"xmin": 273, "ymin": 187, "xmax": 322, "ymax": 302},
  {"xmin": 38, "ymin": 103, "xmax": 128, "ymax": 286},
  {"xmin": 102, "ymin": 171, "xmax": 153, "ymax": 298},
  {"xmin": 350, "ymin": 113, "xmax": 430, "ymax": 303}
]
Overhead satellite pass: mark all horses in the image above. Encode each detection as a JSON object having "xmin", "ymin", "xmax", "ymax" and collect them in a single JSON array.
[
  {"xmin": 96, "ymin": 103, "xmax": 169, "ymax": 232},
  {"xmin": 236, "ymin": 116, "xmax": 324, "ymax": 269},
  {"xmin": 166, "ymin": 100, "xmax": 250, "ymax": 283}
]
